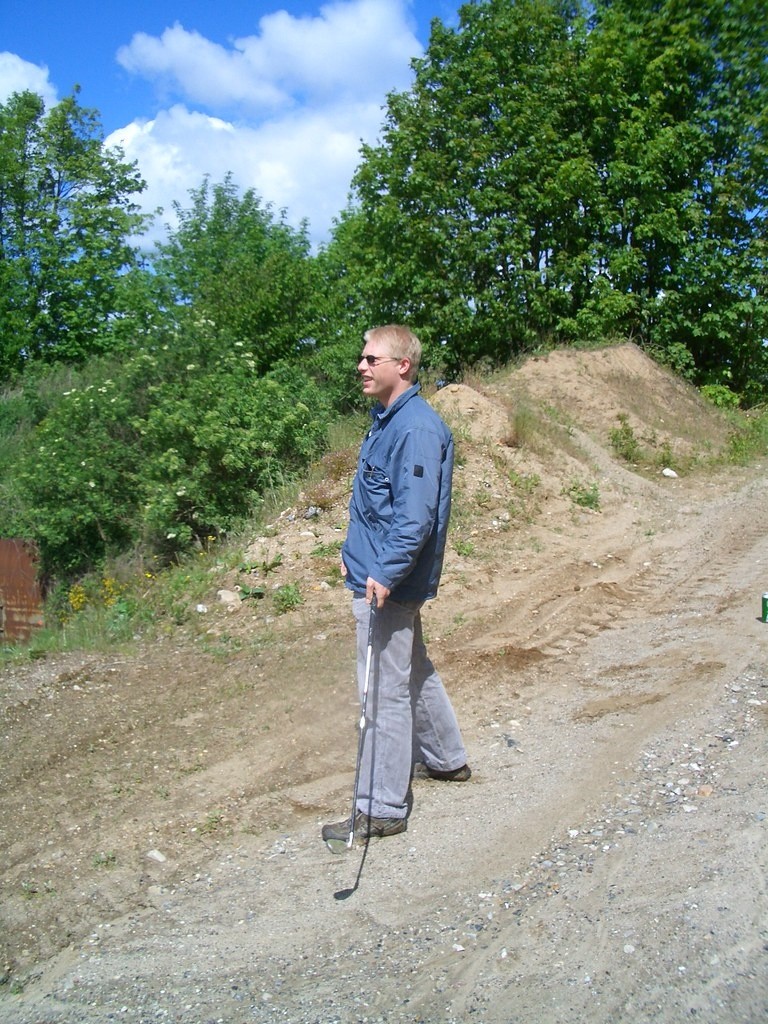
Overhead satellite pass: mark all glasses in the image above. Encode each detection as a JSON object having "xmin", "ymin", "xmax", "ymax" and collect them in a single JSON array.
[{"xmin": 358, "ymin": 355, "xmax": 401, "ymax": 365}]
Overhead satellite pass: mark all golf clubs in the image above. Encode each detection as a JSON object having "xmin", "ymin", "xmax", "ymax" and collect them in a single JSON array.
[{"xmin": 325, "ymin": 593, "xmax": 380, "ymax": 855}]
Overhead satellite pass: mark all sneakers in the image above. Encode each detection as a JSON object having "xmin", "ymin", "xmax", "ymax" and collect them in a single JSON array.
[
  {"xmin": 322, "ymin": 812, "xmax": 407, "ymax": 841},
  {"xmin": 411, "ymin": 762, "xmax": 471, "ymax": 781}
]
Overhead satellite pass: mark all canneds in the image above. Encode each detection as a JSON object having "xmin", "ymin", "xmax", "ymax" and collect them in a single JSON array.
[{"xmin": 761, "ymin": 592, "xmax": 768, "ymax": 623}]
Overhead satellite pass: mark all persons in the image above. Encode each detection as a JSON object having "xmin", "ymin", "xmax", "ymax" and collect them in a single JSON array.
[{"xmin": 321, "ymin": 323, "xmax": 472, "ymax": 839}]
